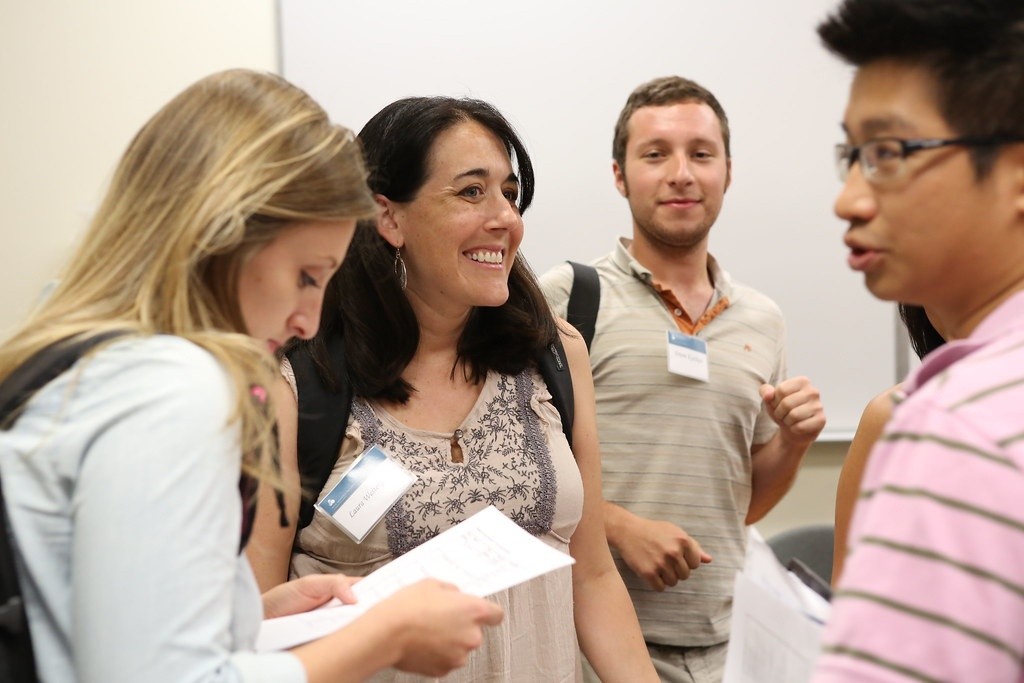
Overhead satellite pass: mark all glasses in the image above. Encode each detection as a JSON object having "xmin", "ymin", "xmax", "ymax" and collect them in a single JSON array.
[{"xmin": 834, "ymin": 135, "xmax": 1022, "ymax": 185}]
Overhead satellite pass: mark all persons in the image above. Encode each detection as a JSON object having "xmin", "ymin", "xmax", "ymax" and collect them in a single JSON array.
[
  {"xmin": 815, "ymin": 0, "xmax": 1023, "ymax": 683},
  {"xmin": 530, "ymin": 74, "xmax": 825, "ymax": 683},
  {"xmin": 828, "ymin": 302, "xmax": 946, "ymax": 598},
  {"xmin": 244, "ymin": 95, "xmax": 666, "ymax": 683},
  {"xmin": 0, "ymin": 66, "xmax": 505, "ymax": 683}
]
